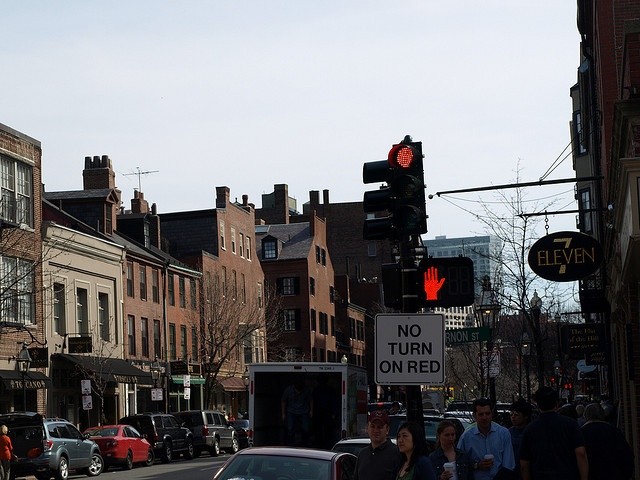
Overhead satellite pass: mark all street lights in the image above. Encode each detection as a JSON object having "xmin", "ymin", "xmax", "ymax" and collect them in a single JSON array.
[
  {"xmin": 555, "ymin": 310, "xmax": 561, "ymax": 361},
  {"xmin": 242, "ymin": 366, "xmax": 250, "ymax": 420},
  {"xmin": 151, "ymin": 354, "xmax": 163, "ymax": 413},
  {"xmin": 470, "ymin": 275, "xmax": 503, "ymax": 400},
  {"xmin": 16, "ymin": 339, "xmax": 32, "ymax": 412},
  {"xmin": 519, "ymin": 327, "xmax": 533, "ymax": 405},
  {"xmin": 531, "ymin": 289, "xmax": 544, "ymax": 386}
]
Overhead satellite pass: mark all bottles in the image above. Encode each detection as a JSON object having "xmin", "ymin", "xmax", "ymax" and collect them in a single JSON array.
[
  {"xmin": 444, "ymin": 463, "xmax": 454, "ymax": 473},
  {"xmin": 484, "ymin": 454, "xmax": 495, "ymax": 463}
]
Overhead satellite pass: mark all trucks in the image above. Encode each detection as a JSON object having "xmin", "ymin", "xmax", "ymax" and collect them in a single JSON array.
[{"xmin": 243, "ymin": 362, "xmax": 368, "ymax": 450}]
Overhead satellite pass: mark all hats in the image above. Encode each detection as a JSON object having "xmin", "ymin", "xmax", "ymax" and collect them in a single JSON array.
[{"xmin": 369, "ymin": 410, "xmax": 389, "ymax": 424}]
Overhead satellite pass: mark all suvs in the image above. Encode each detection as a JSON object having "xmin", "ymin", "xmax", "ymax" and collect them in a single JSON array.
[
  {"xmin": 1, "ymin": 411, "xmax": 104, "ymax": 480},
  {"xmin": 446, "ymin": 400, "xmax": 475, "ymax": 410},
  {"xmin": 119, "ymin": 411, "xmax": 195, "ymax": 463},
  {"xmin": 388, "ymin": 414, "xmax": 447, "ymax": 452}
]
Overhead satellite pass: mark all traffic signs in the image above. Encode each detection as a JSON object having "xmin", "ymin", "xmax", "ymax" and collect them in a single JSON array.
[
  {"xmin": 476, "ymin": 350, "xmax": 500, "ymax": 378},
  {"xmin": 372, "ymin": 312, "xmax": 446, "ymax": 386}
]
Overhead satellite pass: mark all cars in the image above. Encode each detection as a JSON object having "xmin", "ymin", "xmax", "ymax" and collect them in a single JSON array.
[
  {"xmin": 332, "ymin": 438, "xmax": 397, "ymax": 456},
  {"xmin": 444, "ymin": 412, "xmax": 473, "ymax": 418},
  {"xmin": 212, "ymin": 446, "xmax": 358, "ymax": 480},
  {"xmin": 84, "ymin": 424, "xmax": 155, "ymax": 471},
  {"xmin": 229, "ymin": 420, "xmax": 249, "ymax": 448}
]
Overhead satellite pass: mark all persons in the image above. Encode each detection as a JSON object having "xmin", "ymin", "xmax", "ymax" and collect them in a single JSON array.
[
  {"xmin": 353, "ymin": 411, "xmax": 406, "ymax": 480},
  {"xmin": 456, "ymin": 397, "xmax": 516, "ymax": 480},
  {"xmin": 518, "ymin": 387, "xmax": 589, "ymax": 480},
  {"xmin": 506, "ymin": 401, "xmax": 533, "ymax": 458},
  {"xmin": 577, "ymin": 396, "xmax": 614, "ymax": 415},
  {"xmin": 422, "ymin": 423, "xmax": 476, "ymax": 480},
  {"xmin": 281, "ymin": 385, "xmax": 313, "ymax": 446},
  {"xmin": 558, "ymin": 401, "xmax": 578, "ymax": 420},
  {"xmin": 579, "ymin": 403, "xmax": 635, "ymax": 480},
  {"xmin": 388, "ymin": 423, "xmax": 438, "ymax": 480},
  {"xmin": 0, "ymin": 425, "xmax": 12, "ymax": 480}
]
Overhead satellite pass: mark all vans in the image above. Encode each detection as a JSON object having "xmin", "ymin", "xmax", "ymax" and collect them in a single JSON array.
[{"xmin": 173, "ymin": 410, "xmax": 240, "ymax": 456}]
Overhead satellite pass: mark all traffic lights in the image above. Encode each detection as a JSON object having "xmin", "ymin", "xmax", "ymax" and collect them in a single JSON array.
[
  {"xmin": 387, "ymin": 140, "xmax": 428, "ymax": 236},
  {"xmin": 569, "ymin": 382, "xmax": 574, "ymax": 390},
  {"xmin": 362, "ymin": 159, "xmax": 387, "ymax": 242},
  {"xmin": 417, "ymin": 256, "xmax": 474, "ymax": 307},
  {"xmin": 562, "ymin": 381, "xmax": 569, "ymax": 390},
  {"xmin": 549, "ymin": 373, "xmax": 558, "ymax": 384}
]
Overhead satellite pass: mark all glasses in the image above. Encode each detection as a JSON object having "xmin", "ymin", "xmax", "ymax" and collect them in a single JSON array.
[
  {"xmin": 477, "ymin": 410, "xmax": 492, "ymax": 415},
  {"xmin": 510, "ymin": 411, "xmax": 519, "ymax": 416}
]
